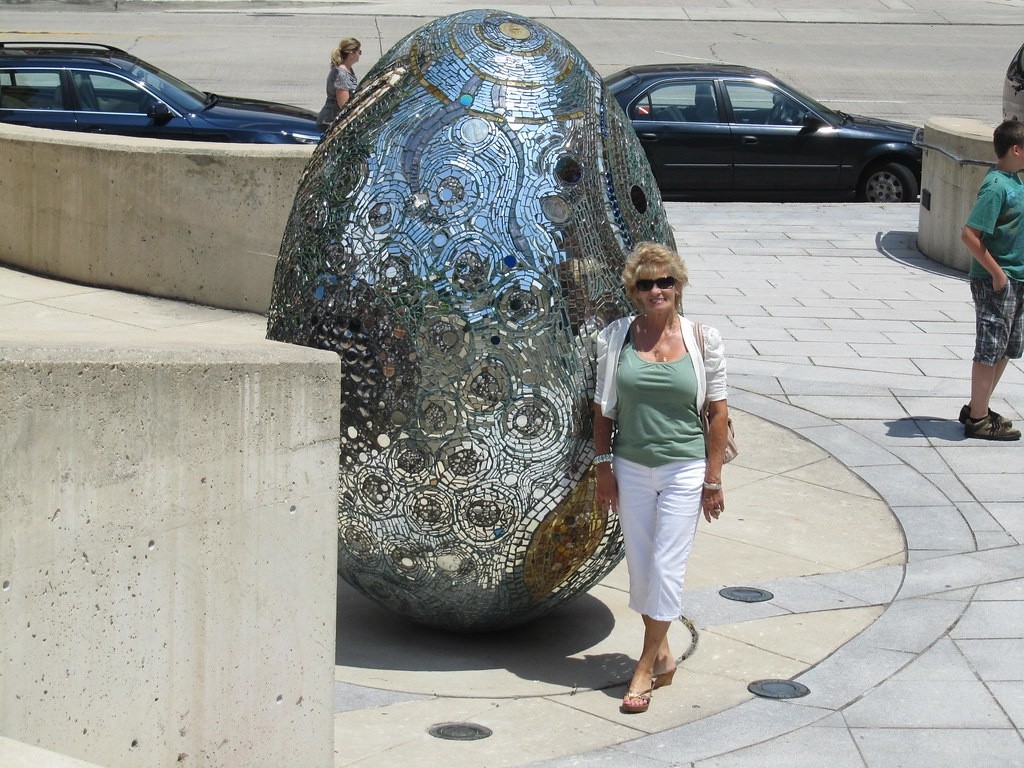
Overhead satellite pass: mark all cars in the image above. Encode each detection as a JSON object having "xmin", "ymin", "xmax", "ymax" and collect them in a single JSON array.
[{"xmin": 601, "ymin": 63, "xmax": 925, "ymax": 203}]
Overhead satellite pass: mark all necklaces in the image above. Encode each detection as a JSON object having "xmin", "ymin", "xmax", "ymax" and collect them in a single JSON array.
[{"xmin": 641, "ymin": 312, "xmax": 678, "ymax": 357}]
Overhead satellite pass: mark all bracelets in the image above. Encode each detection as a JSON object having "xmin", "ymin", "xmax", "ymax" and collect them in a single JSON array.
[
  {"xmin": 593, "ymin": 453, "xmax": 614, "ymax": 465},
  {"xmin": 704, "ymin": 481, "xmax": 722, "ymax": 490}
]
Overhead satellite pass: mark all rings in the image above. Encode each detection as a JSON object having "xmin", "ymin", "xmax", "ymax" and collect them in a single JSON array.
[{"xmin": 715, "ymin": 509, "xmax": 720, "ymax": 513}]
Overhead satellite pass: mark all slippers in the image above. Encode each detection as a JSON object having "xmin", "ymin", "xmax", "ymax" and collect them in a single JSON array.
[
  {"xmin": 622, "ymin": 679, "xmax": 653, "ymax": 712},
  {"xmin": 654, "ymin": 668, "xmax": 678, "ymax": 689}
]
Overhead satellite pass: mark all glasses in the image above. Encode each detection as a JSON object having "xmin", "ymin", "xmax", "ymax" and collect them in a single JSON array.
[
  {"xmin": 358, "ymin": 50, "xmax": 362, "ymax": 55},
  {"xmin": 636, "ymin": 276, "xmax": 676, "ymax": 291}
]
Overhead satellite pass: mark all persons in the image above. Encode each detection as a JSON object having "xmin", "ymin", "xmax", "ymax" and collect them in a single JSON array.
[
  {"xmin": 316, "ymin": 38, "xmax": 362, "ymax": 133},
  {"xmin": 958, "ymin": 120, "xmax": 1024, "ymax": 441},
  {"xmin": 594, "ymin": 243, "xmax": 729, "ymax": 711}
]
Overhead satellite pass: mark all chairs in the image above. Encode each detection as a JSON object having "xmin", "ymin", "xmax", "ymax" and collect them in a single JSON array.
[{"xmin": 695, "ymin": 87, "xmax": 717, "ymax": 122}]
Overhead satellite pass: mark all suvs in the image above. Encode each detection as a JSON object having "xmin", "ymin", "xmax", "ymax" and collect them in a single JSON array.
[{"xmin": 0, "ymin": 42, "xmax": 323, "ymax": 145}]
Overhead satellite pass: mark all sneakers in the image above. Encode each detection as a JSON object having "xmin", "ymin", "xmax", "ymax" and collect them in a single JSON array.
[
  {"xmin": 965, "ymin": 416, "xmax": 1021, "ymax": 442},
  {"xmin": 959, "ymin": 404, "xmax": 1013, "ymax": 427}
]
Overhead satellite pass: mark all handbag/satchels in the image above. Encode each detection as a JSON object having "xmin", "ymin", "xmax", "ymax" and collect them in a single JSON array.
[{"xmin": 700, "ymin": 403, "xmax": 739, "ymax": 465}]
